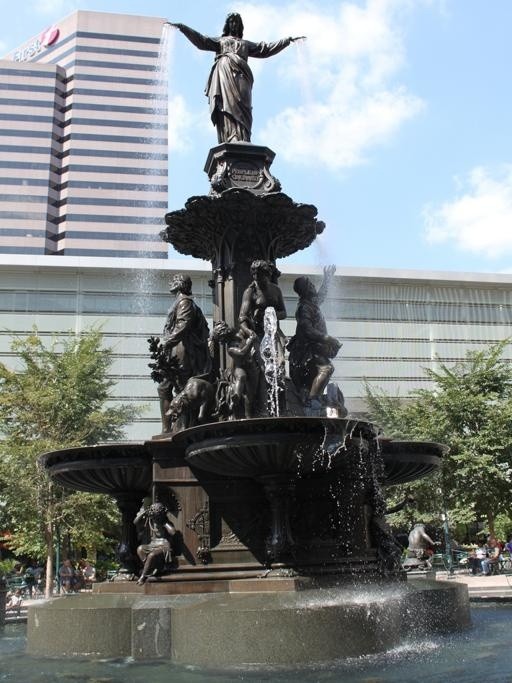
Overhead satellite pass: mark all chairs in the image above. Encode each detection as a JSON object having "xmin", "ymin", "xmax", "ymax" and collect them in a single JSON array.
[{"xmin": 5, "ymin": 568, "xmax": 91, "ymax": 600}]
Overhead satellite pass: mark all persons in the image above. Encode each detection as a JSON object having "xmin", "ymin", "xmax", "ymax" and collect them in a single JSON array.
[
  {"xmin": 59, "ymin": 558, "xmax": 97, "ymax": 592},
  {"xmin": 163, "ymin": 12, "xmax": 306, "ymax": 144},
  {"xmin": 467, "ymin": 535, "xmax": 512, "ymax": 575},
  {"xmin": 408, "ymin": 523, "xmax": 442, "ymax": 558},
  {"xmin": 148, "ymin": 260, "xmax": 341, "ymax": 433},
  {"xmin": 0, "ymin": 557, "xmax": 45, "ymax": 614},
  {"xmin": 133, "ymin": 502, "xmax": 176, "ymax": 586}
]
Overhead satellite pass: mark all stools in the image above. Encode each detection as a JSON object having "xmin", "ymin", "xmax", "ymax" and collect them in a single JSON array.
[{"xmin": 478, "ymin": 558, "xmax": 510, "ymax": 575}]
